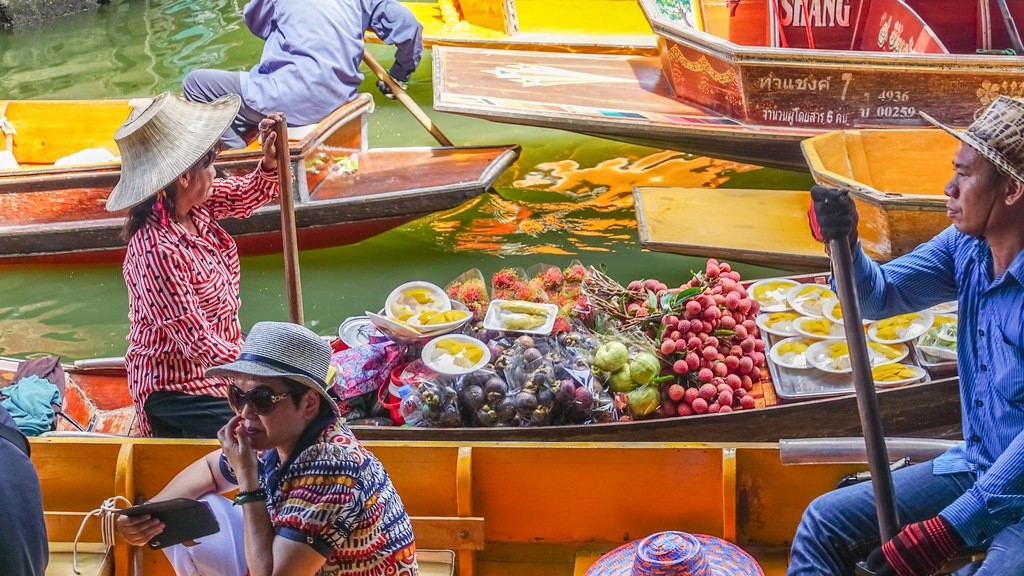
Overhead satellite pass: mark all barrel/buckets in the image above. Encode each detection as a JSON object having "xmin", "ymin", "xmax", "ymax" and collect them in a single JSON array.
[{"xmin": 377, "ymin": 360, "xmax": 436, "ymax": 426}]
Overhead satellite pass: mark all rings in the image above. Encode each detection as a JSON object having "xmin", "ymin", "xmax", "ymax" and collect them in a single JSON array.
[{"xmin": 258, "ymin": 122, "xmax": 269, "ymax": 131}]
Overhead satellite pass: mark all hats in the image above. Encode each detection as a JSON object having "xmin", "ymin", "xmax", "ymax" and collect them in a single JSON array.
[
  {"xmin": 205, "ymin": 320, "xmax": 342, "ymax": 418},
  {"xmin": 920, "ymin": 96, "xmax": 1024, "ymax": 183},
  {"xmin": 586, "ymin": 530, "xmax": 765, "ymax": 576},
  {"xmin": 106, "ymin": 91, "xmax": 241, "ymax": 208}
]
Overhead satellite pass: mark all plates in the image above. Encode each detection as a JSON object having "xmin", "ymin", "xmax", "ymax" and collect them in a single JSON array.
[
  {"xmin": 746, "ymin": 277, "xmax": 959, "ymax": 388},
  {"xmin": 338, "ymin": 280, "xmax": 491, "ymax": 375},
  {"xmin": 483, "ymin": 299, "xmax": 559, "ymax": 336}
]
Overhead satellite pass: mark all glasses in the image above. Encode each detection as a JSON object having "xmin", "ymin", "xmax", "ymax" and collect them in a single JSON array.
[{"xmin": 227, "ymin": 385, "xmax": 295, "ymax": 415}]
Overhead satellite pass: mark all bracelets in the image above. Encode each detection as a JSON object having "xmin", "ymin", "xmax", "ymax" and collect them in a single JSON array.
[
  {"xmin": 232, "ymin": 487, "xmax": 265, "ymax": 506},
  {"xmin": 234, "ymin": 493, "xmax": 268, "ymax": 506}
]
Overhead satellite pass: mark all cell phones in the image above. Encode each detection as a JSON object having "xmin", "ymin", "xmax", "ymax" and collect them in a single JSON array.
[{"xmin": 118, "ymin": 498, "xmax": 221, "ymax": 551}]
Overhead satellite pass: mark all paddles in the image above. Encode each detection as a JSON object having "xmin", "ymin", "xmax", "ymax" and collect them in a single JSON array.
[
  {"xmin": 276, "ymin": 116, "xmax": 305, "ymax": 327},
  {"xmin": 827, "ymin": 231, "xmax": 901, "ymax": 540},
  {"xmin": 361, "ymin": 50, "xmax": 500, "ymax": 198}
]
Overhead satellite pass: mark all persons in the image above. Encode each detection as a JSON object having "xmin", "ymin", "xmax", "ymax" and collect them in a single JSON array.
[
  {"xmin": 105, "ymin": 91, "xmax": 285, "ymax": 438},
  {"xmin": 181, "ymin": 0, "xmax": 423, "ymax": 152},
  {"xmin": 116, "ymin": 320, "xmax": 422, "ymax": 576},
  {"xmin": 785, "ymin": 96, "xmax": 1023, "ymax": 576}
]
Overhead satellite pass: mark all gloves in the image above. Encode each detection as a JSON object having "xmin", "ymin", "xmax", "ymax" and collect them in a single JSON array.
[
  {"xmin": 376, "ymin": 77, "xmax": 408, "ymax": 98},
  {"xmin": 866, "ymin": 516, "xmax": 964, "ymax": 576},
  {"xmin": 808, "ymin": 185, "xmax": 858, "ymax": 251}
]
